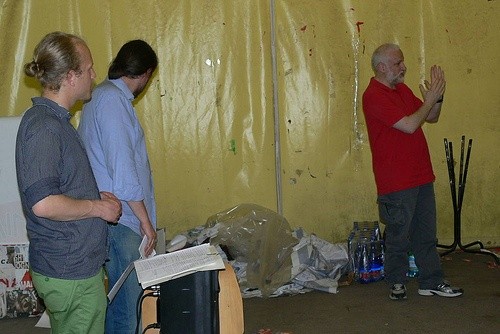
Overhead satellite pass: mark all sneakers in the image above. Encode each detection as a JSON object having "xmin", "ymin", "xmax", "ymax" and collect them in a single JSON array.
[
  {"xmin": 417, "ymin": 282, "xmax": 464, "ymax": 297},
  {"xmin": 389, "ymin": 283, "xmax": 408, "ymax": 300}
]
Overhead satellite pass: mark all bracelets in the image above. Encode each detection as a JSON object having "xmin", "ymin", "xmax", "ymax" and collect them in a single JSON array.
[{"xmin": 437, "ymin": 95, "xmax": 444, "ymax": 103}]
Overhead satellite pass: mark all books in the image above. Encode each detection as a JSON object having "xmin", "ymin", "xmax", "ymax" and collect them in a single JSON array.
[{"xmin": 134, "ymin": 234, "xmax": 225, "ymax": 290}]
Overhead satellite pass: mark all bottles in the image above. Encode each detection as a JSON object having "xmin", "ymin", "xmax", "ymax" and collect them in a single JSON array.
[
  {"xmin": 348, "ymin": 221, "xmax": 386, "ymax": 284},
  {"xmin": 408, "ymin": 255, "xmax": 419, "ymax": 277}
]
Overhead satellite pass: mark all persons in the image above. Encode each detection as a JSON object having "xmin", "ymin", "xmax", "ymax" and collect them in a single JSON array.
[
  {"xmin": 15, "ymin": 29, "xmax": 122, "ymax": 334},
  {"xmin": 77, "ymin": 39, "xmax": 159, "ymax": 334},
  {"xmin": 361, "ymin": 43, "xmax": 463, "ymax": 300}
]
